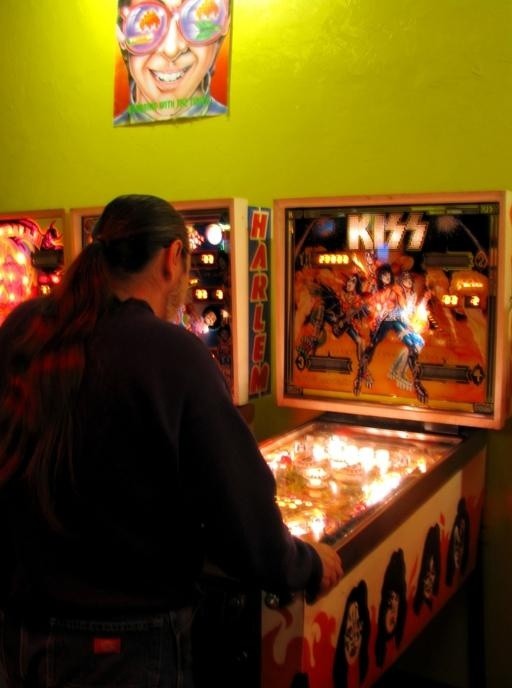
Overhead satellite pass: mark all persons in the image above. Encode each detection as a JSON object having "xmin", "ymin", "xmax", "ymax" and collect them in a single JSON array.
[
  {"xmin": 444, "ymin": 494, "xmax": 471, "ymax": 593},
  {"xmin": 372, "ymin": 547, "xmax": 409, "ymax": 670},
  {"xmin": 331, "ymin": 578, "xmax": 371, "ymax": 687},
  {"xmin": 411, "ymin": 522, "xmax": 442, "ymax": 618},
  {"xmin": 0, "ymin": 193, "xmax": 345, "ymax": 687},
  {"xmin": 116, "ymin": 0, "xmax": 231, "ymax": 129},
  {"xmin": 174, "ymin": 301, "xmax": 232, "ymax": 365},
  {"xmin": 298, "ymin": 251, "xmax": 430, "ymax": 406}
]
